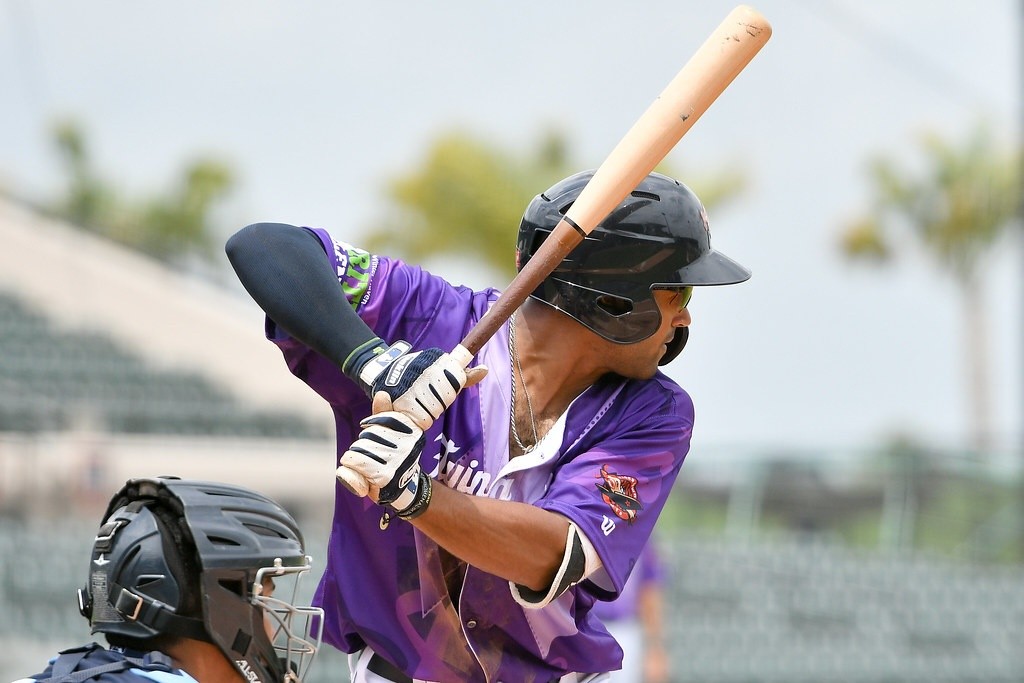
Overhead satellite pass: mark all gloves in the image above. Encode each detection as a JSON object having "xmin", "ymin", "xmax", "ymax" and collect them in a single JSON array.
[
  {"xmin": 357, "ymin": 339, "xmax": 490, "ymax": 433},
  {"xmin": 339, "ymin": 391, "xmax": 427, "ymax": 513}
]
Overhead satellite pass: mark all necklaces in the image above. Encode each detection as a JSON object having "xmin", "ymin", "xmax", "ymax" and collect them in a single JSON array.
[{"xmin": 507, "ymin": 310, "xmax": 548, "ymax": 455}]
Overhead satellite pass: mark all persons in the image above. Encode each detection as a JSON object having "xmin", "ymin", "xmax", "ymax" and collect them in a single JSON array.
[
  {"xmin": 223, "ymin": 164, "xmax": 752, "ymax": 683},
  {"xmin": 11, "ymin": 477, "xmax": 321, "ymax": 683}
]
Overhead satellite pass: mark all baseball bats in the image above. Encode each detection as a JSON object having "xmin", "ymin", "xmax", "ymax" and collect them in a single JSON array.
[{"xmin": 330, "ymin": 2, "xmax": 775, "ymax": 502}]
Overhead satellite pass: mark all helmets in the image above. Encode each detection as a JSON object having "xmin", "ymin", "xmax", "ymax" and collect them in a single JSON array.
[
  {"xmin": 514, "ymin": 167, "xmax": 753, "ymax": 368},
  {"xmin": 76, "ymin": 475, "xmax": 307, "ymax": 683}
]
